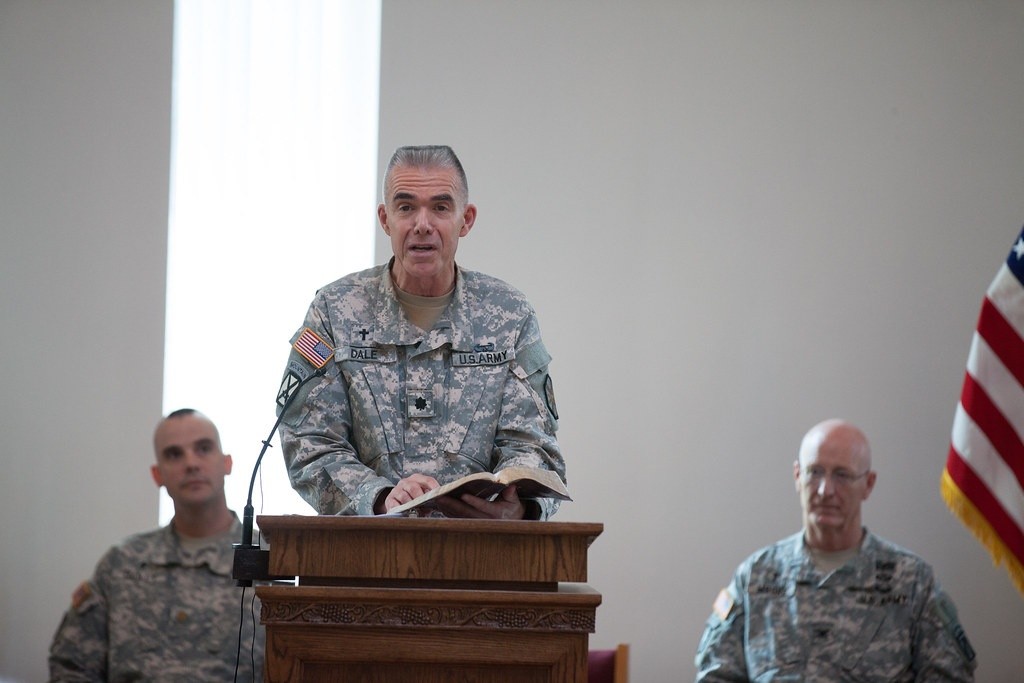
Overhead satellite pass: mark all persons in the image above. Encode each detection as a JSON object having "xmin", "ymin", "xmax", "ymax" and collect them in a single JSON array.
[
  {"xmin": 694, "ymin": 419, "xmax": 978, "ymax": 683},
  {"xmin": 276, "ymin": 145, "xmax": 568, "ymax": 521},
  {"xmin": 47, "ymin": 408, "xmax": 271, "ymax": 683}
]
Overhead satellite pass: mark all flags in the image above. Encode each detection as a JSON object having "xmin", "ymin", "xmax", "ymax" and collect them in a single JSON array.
[{"xmin": 941, "ymin": 227, "xmax": 1024, "ymax": 599}]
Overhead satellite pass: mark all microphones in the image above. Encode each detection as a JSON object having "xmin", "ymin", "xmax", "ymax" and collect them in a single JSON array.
[{"xmin": 231, "ymin": 367, "xmax": 327, "ymax": 549}]
